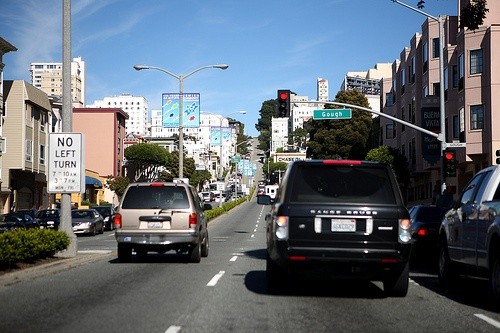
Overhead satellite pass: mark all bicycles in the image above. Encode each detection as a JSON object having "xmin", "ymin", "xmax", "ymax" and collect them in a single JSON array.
[{"xmin": 439, "ymin": 164, "xmax": 500, "ymax": 304}]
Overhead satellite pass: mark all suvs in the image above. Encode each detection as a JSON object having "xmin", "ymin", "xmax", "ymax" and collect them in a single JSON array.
[
  {"xmin": 112, "ymin": 180, "xmax": 211, "ymax": 264},
  {"xmin": 405, "ymin": 203, "xmax": 451, "ymax": 258},
  {"xmin": 268, "ymin": 159, "xmax": 414, "ymax": 298}
]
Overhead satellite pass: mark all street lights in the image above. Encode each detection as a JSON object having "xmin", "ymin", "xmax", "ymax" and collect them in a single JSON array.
[
  {"xmin": 133, "ymin": 63, "xmax": 229, "ymax": 183},
  {"xmin": 200, "ymin": 109, "xmax": 247, "ymax": 209}
]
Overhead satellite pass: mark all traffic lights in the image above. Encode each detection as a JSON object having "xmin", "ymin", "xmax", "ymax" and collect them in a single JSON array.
[
  {"xmin": 496, "ymin": 149, "xmax": 500, "ymax": 164},
  {"xmin": 443, "ymin": 149, "xmax": 457, "ymax": 178},
  {"xmin": 277, "ymin": 89, "xmax": 290, "ymax": 117}
]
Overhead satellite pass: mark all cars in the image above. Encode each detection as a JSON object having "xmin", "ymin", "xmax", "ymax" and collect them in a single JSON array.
[
  {"xmin": 196, "ymin": 171, "xmax": 248, "ymax": 210},
  {"xmin": 90, "ymin": 206, "xmax": 118, "ymax": 232},
  {"xmin": 256, "ymin": 180, "xmax": 279, "ymax": 203},
  {"xmin": 0, "ymin": 208, "xmax": 59, "ymax": 233},
  {"xmin": 71, "ymin": 209, "xmax": 106, "ymax": 236}
]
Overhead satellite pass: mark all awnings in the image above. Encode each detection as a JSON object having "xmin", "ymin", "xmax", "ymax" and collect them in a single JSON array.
[{"xmin": 85, "ymin": 177, "xmax": 104, "ymax": 187}]
